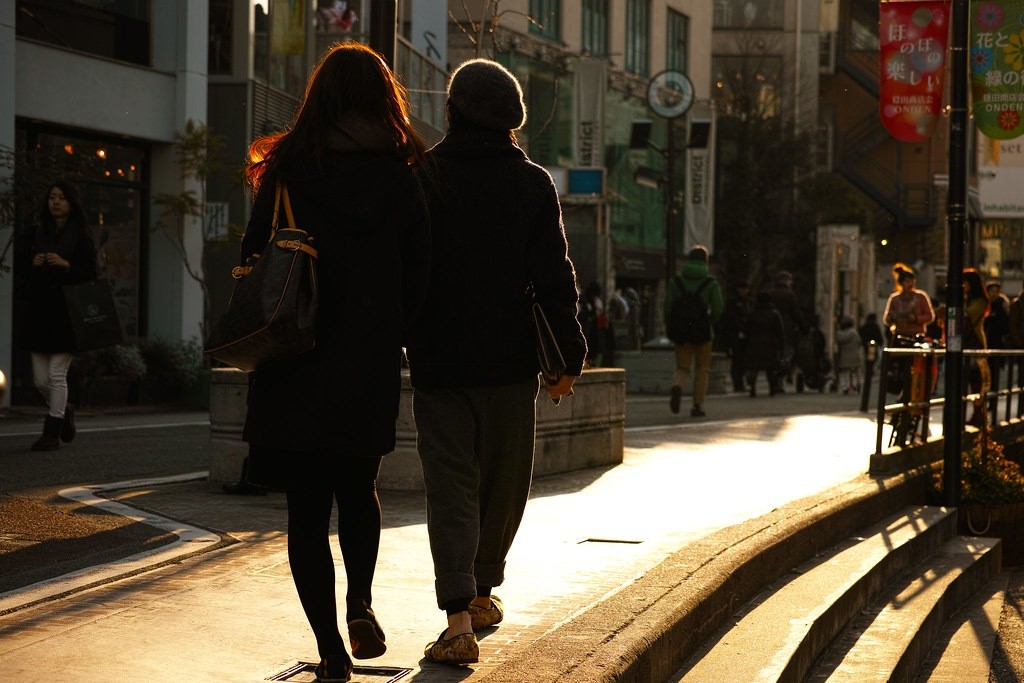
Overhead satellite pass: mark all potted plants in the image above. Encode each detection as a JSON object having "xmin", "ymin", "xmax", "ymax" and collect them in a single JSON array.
[
  {"xmin": 141, "ymin": 119, "xmax": 249, "ymax": 412},
  {"xmin": 78, "ymin": 344, "xmax": 142, "ymax": 409}
]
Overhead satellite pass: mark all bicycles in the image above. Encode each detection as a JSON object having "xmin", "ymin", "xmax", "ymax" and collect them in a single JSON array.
[{"xmin": 887, "ymin": 331, "xmax": 948, "ymax": 447}]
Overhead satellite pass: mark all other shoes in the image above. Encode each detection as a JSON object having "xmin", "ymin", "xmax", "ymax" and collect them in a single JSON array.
[
  {"xmin": 424, "ymin": 626, "xmax": 480, "ymax": 665},
  {"xmin": 670, "ymin": 385, "xmax": 681, "ymax": 413},
  {"xmin": 691, "ymin": 408, "xmax": 705, "ymax": 417},
  {"xmin": 470, "ymin": 595, "xmax": 504, "ymax": 631},
  {"xmin": 316, "ymin": 653, "xmax": 354, "ymax": 683},
  {"xmin": 966, "ymin": 411, "xmax": 981, "ymax": 424},
  {"xmin": 347, "ymin": 599, "xmax": 387, "ymax": 659}
]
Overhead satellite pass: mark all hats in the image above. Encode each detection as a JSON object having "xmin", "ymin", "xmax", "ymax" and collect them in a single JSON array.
[{"xmin": 450, "ymin": 59, "xmax": 526, "ymax": 130}]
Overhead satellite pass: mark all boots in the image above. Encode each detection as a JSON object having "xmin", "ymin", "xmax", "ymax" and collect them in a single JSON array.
[
  {"xmin": 32, "ymin": 414, "xmax": 65, "ymax": 450},
  {"xmin": 61, "ymin": 402, "xmax": 76, "ymax": 442}
]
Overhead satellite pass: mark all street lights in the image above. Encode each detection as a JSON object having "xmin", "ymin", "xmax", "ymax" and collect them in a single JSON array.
[{"xmin": 626, "ymin": 67, "xmax": 714, "ymax": 286}]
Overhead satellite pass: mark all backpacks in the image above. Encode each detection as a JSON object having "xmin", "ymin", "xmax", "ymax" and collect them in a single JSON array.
[{"xmin": 675, "ymin": 275, "xmax": 713, "ymax": 341}]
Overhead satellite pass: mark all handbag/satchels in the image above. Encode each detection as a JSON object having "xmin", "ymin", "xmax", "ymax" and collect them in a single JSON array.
[
  {"xmin": 59, "ymin": 278, "xmax": 123, "ymax": 352},
  {"xmin": 202, "ymin": 179, "xmax": 317, "ymax": 370},
  {"xmin": 531, "ymin": 298, "xmax": 574, "ymax": 406}
]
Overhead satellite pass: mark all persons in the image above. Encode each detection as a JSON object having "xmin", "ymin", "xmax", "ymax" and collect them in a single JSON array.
[
  {"xmin": 860, "ymin": 314, "xmax": 883, "ymax": 373},
  {"xmin": 964, "ymin": 269, "xmax": 990, "ymax": 423},
  {"xmin": 668, "ymin": 247, "xmax": 724, "ymax": 416},
  {"xmin": 983, "ymin": 281, "xmax": 1009, "ymax": 412},
  {"xmin": 835, "ymin": 318, "xmax": 862, "ymax": 393},
  {"xmin": 611, "ymin": 288, "xmax": 629, "ymax": 315},
  {"xmin": 724, "ymin": 272, "xmax": 831, "ymax": 397},
  {"xmin": 242, "ymin": 46, "xmax": 424, "ymax": 680},
  {"xmin": 19, "ymin": 182, "xmax": 98, "ymax": 448},
  {"xmin": 406, "ymin": 58, "xmax": 586, "ymax": 663},
  {"xmin": 883, "ymin": 265, "xmax": 934, "ymax": 401},
  {"xmin": 1009, "ymin": 290, "xmax": 1024, "ymax": 389}
]
